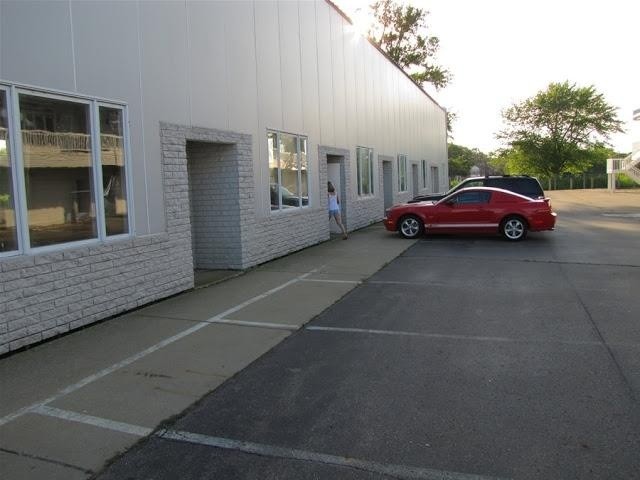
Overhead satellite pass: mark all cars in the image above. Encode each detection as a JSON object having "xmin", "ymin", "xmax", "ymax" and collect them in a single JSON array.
[
  {"xmin": 270, "ymin": 181, "xmax": 309, "ymax": 206},
  {"xmin": 383, "ymin": 186, "xmax": 556, "ymax": 241}
]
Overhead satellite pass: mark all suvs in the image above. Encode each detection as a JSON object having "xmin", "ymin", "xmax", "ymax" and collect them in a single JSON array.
[{"xmin": 408, "ymin": 174, "xmax": 545, "ymax": 204}]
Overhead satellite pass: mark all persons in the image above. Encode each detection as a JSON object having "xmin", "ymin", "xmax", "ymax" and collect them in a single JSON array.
[{"xmin": 327, "ymin": 181, "xmax": 349, "ymax": 241}]
